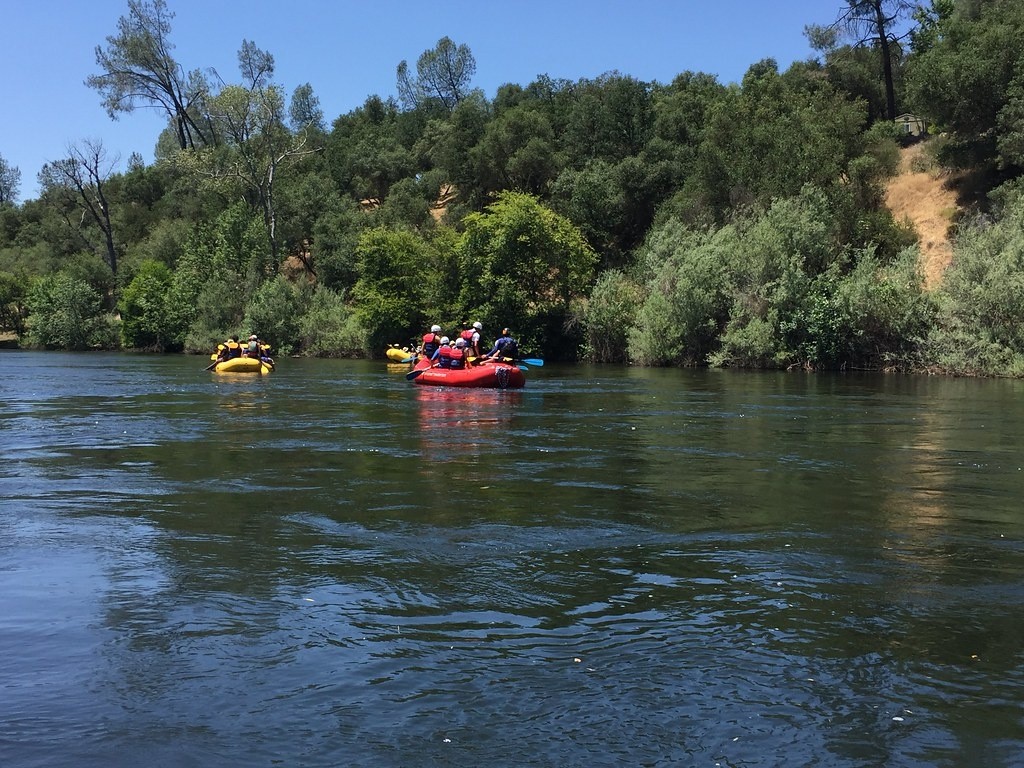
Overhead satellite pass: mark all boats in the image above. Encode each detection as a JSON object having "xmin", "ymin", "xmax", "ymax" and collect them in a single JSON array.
[
  {"xmin": 386, "ymin": 349, "xmax": 422, "ymax": 363},
  {"xmin": 215, "ymin": 358, "xmax": 272, "ymax": 374},
  {"xmin": 413, "ymin": 356, "xmax": 526, "ymax": 388}
]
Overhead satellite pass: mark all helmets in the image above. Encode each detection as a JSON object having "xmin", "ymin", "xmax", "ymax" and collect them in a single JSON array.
[{"xmin": 253, "ymin": 335, "xmax": 258, "ymax": 340}]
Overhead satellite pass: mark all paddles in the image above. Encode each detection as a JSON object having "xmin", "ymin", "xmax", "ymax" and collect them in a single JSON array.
[
  {"xmin": 486, "ymin": 355, "xmax": 544, "ymax": 370},
  {"xmin": 401, "ymin": 356, "xmax": 418, "ymax": 363},
  {"xmin": 200, "ymin": 359, "xmax": 220, "ymax": 371},
  {"xmin": 406, "ymin": 362, "xmax": 439, "ymax": 380}
]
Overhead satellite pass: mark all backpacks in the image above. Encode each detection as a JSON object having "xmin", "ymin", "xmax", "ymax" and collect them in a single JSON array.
[{"xmin": 503, "ymin": 341, "xmax": 516, "ymax": 356}]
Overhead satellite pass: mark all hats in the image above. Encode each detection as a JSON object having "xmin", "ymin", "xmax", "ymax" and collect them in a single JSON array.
[
  {"xmin": 224, "ymin": 339, "xmax": 238, "ymax": 349},
  {"xmin": 217, "ymin": 344, "xmax": 224, "ymax": 351},
  {"xmin": 431, "ymin": 325, "xmax": 442, "ymax": 333},
  {"xmin": 473, "ymin": 322, "xmax": 482, "ymax": 330},
  {"xmin": 264, "ymin": 344, "xmax": 271, "ymax": 349},
  {"xmin": 450, "ymin": 340, "xmax": 455, "ymax": 346},
  {"xmin": 504, "ymin": 327, "xmax": 511, "ymax": 333},
  {"xmin": 393, "ymin": 344, "xmax": 400, "ymax": 347},
  {"xmin": 403, "ymin": 347, "xmax": 409, "ymax": 351},
  {"xmin": 455, "ymin": 338, "xmax": 466, "ymax": 346},
  {"xmin": 440, "ymin": 336, "xmax": 449, "ymax": 344}
]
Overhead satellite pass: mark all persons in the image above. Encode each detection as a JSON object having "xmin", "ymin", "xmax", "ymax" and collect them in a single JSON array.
[
  {"xmin": 421, "ymin": 321, "xmax": 518, "ymax": 370},
  {"xmin": 216, "ymin": 334, "xmax": 275, "ymax": 364}
]
